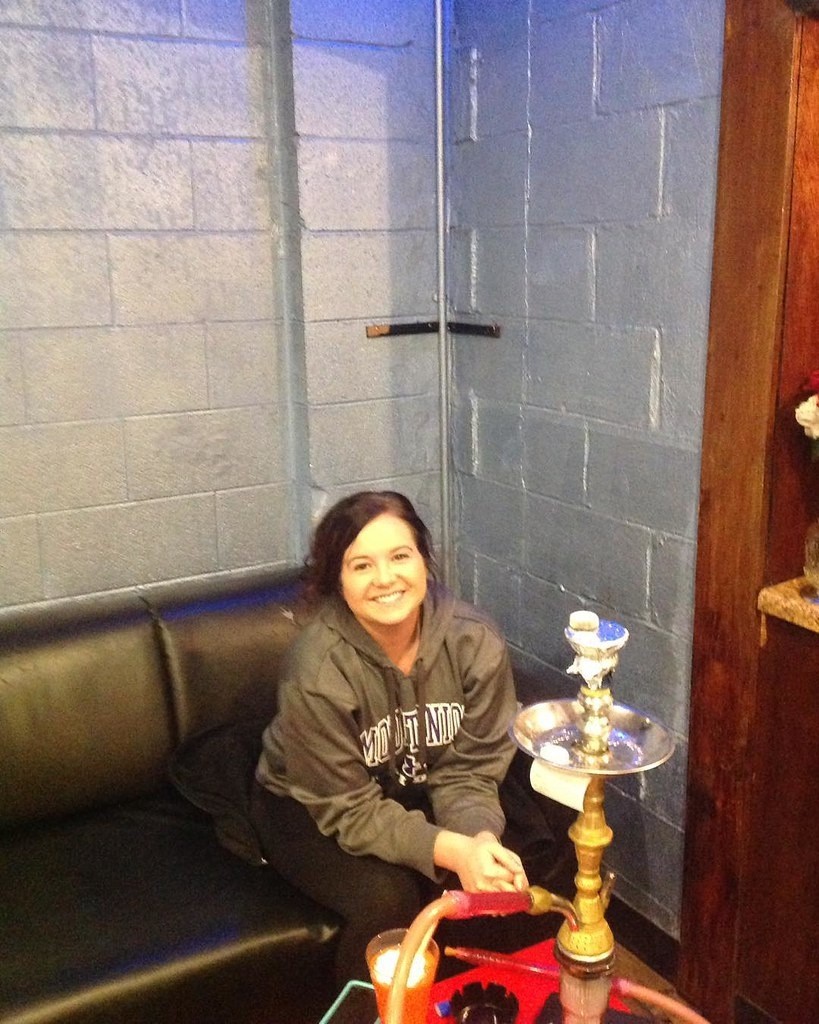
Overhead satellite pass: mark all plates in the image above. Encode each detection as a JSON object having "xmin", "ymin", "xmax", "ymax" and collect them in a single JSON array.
[{"xmin": 507, "ymin": 697, "xmax": 676, "ymax": 775}]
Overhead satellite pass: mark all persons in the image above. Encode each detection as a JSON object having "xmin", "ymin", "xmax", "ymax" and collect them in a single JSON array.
[{"xmin": 250, "ymin": 489, "xmax": 519, "ymax": 1024}]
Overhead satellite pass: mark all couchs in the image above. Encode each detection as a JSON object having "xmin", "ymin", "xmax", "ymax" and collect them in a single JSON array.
[{"xmin": 0, "ymin": 563, "xmax": 346, "ymax": 1024}]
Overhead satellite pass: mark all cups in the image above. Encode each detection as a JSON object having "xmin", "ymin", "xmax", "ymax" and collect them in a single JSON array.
[{"xmin": 365, "ymin": 926, "xmax": 440, "ymax": 1024}]
